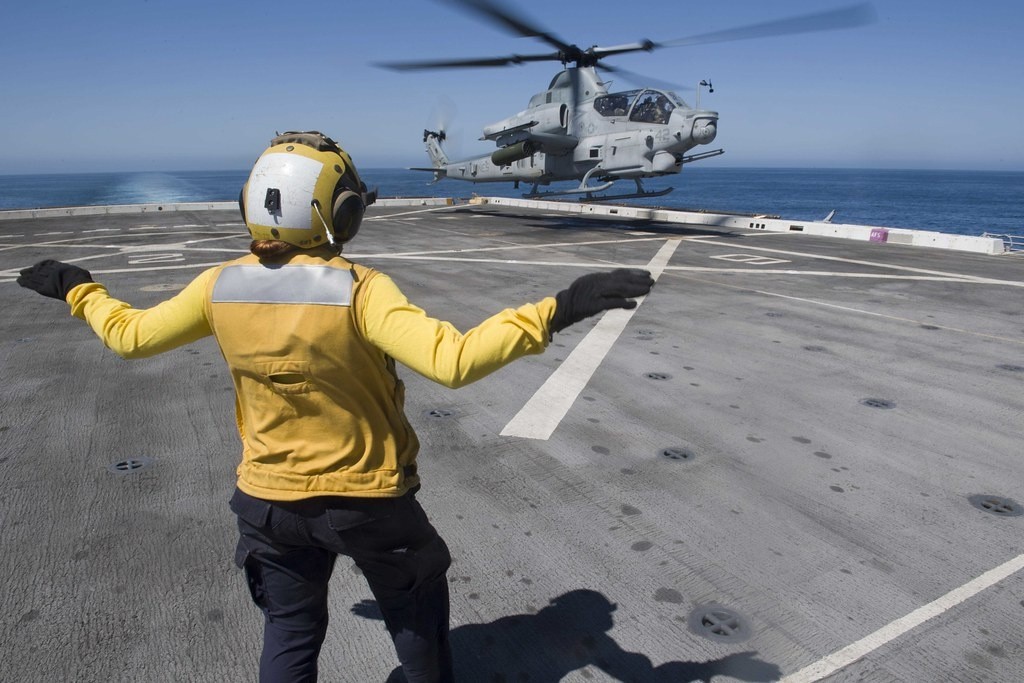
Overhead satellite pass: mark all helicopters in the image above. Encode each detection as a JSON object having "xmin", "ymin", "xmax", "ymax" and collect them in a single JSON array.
[{"xmin": 359, "ymin": 0, "xmax": 897, "ymax": 204}]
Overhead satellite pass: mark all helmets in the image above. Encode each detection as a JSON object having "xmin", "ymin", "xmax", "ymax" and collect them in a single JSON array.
[{"xmin": 239, "ymin": 130, "xmax": 379, "ymax": 249}]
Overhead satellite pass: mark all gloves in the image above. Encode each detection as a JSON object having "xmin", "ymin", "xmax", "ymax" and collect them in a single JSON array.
[
  {"xmin": 16, "ymin": 260, "xmax": 95, "ymax": 302},
  {"xmin": 551, "ymin": 268, "xmax": 655, "ymax": 332}
]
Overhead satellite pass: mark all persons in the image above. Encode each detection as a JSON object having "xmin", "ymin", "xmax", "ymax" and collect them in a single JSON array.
[{"xmin": 17, "ymin": 131, "xmax": 656, "ymax": 683}]
